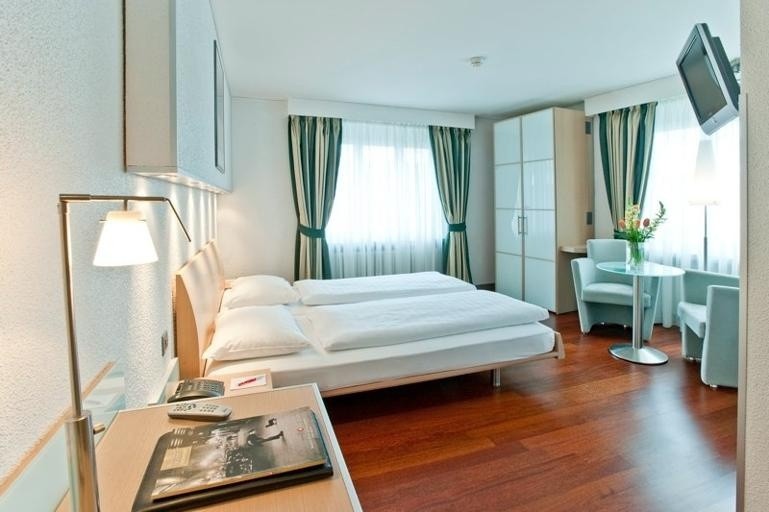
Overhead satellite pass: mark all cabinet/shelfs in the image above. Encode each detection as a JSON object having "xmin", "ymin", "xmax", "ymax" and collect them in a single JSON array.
[{"xmin": 492, "ymin": 106, "xmax": 594, "ymax": 316}]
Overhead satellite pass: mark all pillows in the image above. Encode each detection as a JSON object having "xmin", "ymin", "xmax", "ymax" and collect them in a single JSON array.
[
  {"xmin": 224, "ymin": 274, "xmax": 298, "ymax": 308},
  {"xmin": 202, "ymin": 304, "xmax": 315, "ymax": 362}
]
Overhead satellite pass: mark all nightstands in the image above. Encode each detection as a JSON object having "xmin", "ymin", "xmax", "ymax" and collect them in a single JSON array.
[{"xmin": 166, "ymin": 367, "xmax": 273, "ymax": 403}]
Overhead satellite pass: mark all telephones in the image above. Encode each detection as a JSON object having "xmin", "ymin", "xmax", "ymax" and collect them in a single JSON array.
[{"xmin": 167, "ymin": 380, "xmax": 225, "ymax": 403}]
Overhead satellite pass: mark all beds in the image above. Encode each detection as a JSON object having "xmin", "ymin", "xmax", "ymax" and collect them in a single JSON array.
[{"xmin": 172, "ymin": 240, "xmax": 565, "ymax": 399}]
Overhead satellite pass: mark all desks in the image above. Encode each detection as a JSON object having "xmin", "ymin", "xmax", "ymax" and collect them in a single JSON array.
[
  {"xmin": 596, "ymin": 261, "xmax": 685, "ymax": 365},
  {"xmin": 52, "ymin": 383, "xmax": 362, "ymax": 512}
]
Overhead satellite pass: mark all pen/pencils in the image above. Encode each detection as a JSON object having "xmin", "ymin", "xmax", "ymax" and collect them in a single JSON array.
[{"xmin": 238, "ymin": 376, "xmax": 263, "ymax": 386}]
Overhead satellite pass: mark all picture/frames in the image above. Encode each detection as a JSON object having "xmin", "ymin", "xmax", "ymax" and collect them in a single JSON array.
[{"xmin": 213, "ymin": 38, "xmax": 225, "ymax": 175}]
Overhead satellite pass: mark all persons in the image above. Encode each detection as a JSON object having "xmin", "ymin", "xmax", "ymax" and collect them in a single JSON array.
[{"xmin": 246, "ymin": 430, "xmax": 283, "ymax": 447}]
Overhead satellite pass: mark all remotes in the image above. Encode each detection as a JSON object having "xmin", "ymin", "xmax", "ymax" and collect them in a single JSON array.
[{"xmin": 167, "ymin": 401, "xmax": 233, "ymax": 420}]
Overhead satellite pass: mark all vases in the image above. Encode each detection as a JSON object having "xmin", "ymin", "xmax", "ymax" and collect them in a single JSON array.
[{"xmin": 625, "ymin": 238, "xmax": 645, "ymax": 275}]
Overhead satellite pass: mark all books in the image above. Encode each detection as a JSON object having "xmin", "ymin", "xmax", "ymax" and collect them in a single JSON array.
[
  {"xmin": 131, "ymin": 407, "xmax": 334, "ymax": 512},
  {"xmin": 150, "ymin": 407, "xmax": 327, "ymax": 502}
]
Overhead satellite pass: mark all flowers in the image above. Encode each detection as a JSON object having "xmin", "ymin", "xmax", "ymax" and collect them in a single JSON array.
[{"xmin": 612, "ymin": 195, "xmax": 665, "ymax": 264}]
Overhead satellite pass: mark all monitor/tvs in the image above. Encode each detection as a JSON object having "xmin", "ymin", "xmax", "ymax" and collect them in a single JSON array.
[{"xmin": 676, "ymin": 22, "xmax": 743, "ymax": 137}]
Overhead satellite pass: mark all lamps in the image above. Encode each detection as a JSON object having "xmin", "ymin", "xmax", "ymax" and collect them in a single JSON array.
[
  {"xmin": 57, "ymin": 193, "xmax": 191, "ymax": 511},
  {"xmin": 685, "ymin": 137, "xmax": 723, "ymax": 270}
]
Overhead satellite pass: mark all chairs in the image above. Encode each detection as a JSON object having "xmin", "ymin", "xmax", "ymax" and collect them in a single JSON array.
[
  {"xmin": 677, "ymin": 268, "xmax": 739, "ymax": 389},
  {"xmin": 571, "ymin": 238, "xmax": 664, "ymax": 342}
]
